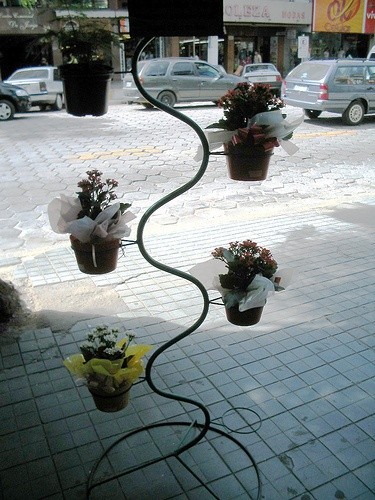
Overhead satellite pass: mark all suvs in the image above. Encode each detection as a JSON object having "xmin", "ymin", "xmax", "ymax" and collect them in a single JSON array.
[{"xmin": 122, "ymin": 57, "xmax": 255, "ymax": 109}]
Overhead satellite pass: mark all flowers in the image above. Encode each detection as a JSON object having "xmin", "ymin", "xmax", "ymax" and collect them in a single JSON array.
[
  {"xmin": 73, "ymin": 170, "xmax": 131, "ymax": 220},
  {"xmin": 203, "ymin": 82, "xmax": 294, "ymax": 129},
  {"xmin": 81, "ymin": 322, "xmax": 134, "ymax": 368},
  {"xmin": 211, "ymin": 240, "xmax": 281, "ymax": 291}
]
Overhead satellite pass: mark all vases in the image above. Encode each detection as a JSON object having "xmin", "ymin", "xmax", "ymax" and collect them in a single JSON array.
[
  {"xmin": 224, "ymin": 142, "xmax": 272, "ymax": 180},
  {"xmin": 224, "ymin": 297, "xmax": 268, "ymax": 325},
  {"xmin": 84, "ymin": 375, "xmax": 134, "ymax": 412},
  {"xmin": 70, "ymin": 237, "xmax": 120, "ymax": 274}
]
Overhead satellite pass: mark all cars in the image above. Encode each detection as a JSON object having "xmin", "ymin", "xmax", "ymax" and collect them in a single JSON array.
[
  {"xmin": 4, "ymin": 66, "xmax": 65, "ymax": 111},
  {"xmin": 240, "ymin": 62, "xmax": 283, "ymax": 96},
  {"xmin": 0, "ymin": 83, "xmax": 30, "ymax": 121}
]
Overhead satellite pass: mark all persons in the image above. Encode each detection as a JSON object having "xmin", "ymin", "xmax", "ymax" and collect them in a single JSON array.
[
  {"xmin": 324, "ymin": 47, "xmax": 330, "ymax": 58},
  {"xmin": 253, "ymin": 50, "xmax": 262, "ymax": 63},
  {"xmin": 336, "ymin": 46, "xmax": 345, "ymax": 58},
  {"xmin": 246, "ymin": 55, "xmax": 252, "ymax": 64},
  {"xmin": 40, "ymin": 58, "xmax": 52, "ymax": 67}
]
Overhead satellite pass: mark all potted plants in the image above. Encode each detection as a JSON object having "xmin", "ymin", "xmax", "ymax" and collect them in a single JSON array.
[{"xmin": 24, "ymin": 0, "xmax": 128, "ymax": 116}]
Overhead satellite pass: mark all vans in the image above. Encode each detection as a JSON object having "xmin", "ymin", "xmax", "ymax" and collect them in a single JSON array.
[{"xmin": 280, "ymin": 59, "xmax": 374, "ymax": 126}]
